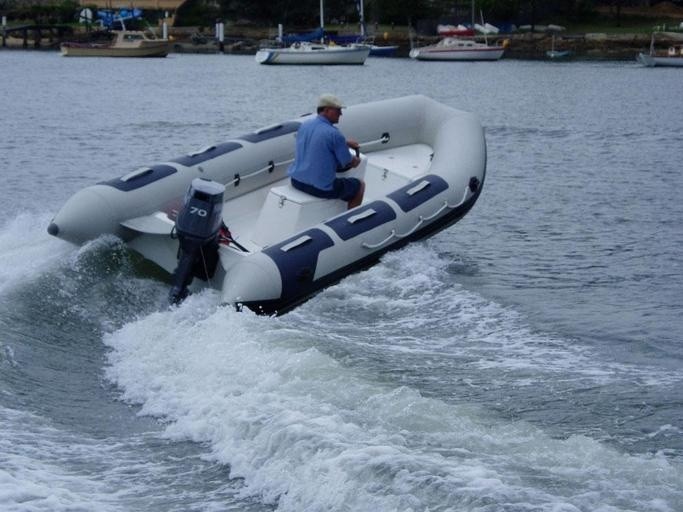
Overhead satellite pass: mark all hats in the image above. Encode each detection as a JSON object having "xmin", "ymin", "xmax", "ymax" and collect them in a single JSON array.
[{"xmin": 317, "ymin": 94, "xmax": 346, "ymax": 109}]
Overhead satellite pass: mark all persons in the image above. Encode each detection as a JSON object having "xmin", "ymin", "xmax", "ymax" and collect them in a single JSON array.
[{"xmin": 288, "ymin": 94, "xmax": 366, "ymax": 211}]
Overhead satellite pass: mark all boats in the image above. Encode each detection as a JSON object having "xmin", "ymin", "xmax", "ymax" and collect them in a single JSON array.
[
  {"xmin": 408, "ymin": 37, "xmax": 510, "ymax": 60},
  {"xmin": 638, "ymin": 46, "xmax": 682, "ymax": 67},
  {"xmin": 46, "ymin": 94, "xmax": 486, "ymax": 316},
  {"xmin": 346, "ymin": 41, "xmax": 401, "ymax": 57},
  {"xmin": 255, "ymin": 41, "xmax": 372, "ymax": 65},
  {"xmin": 59, "ymin": 28, "xmax": 168, "ymax": 57}
]
